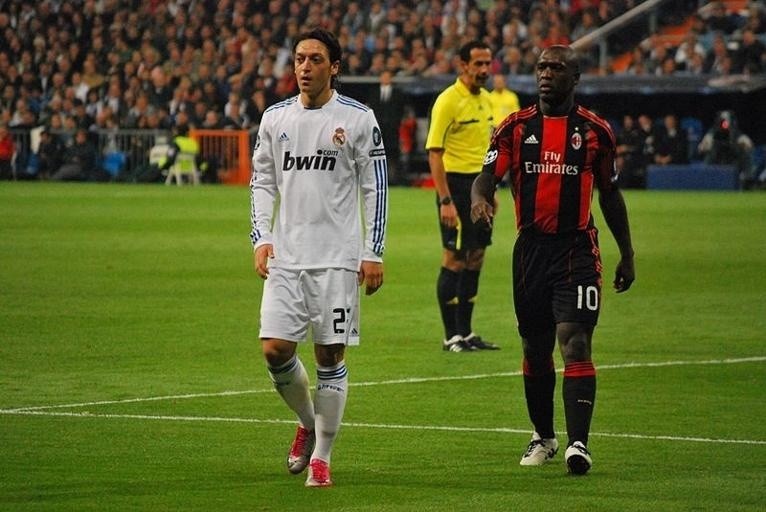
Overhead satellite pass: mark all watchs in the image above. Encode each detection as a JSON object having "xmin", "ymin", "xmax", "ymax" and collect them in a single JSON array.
[{"xmin": 437, "ymin": 195, "xmax": 453, "ymax": 208}]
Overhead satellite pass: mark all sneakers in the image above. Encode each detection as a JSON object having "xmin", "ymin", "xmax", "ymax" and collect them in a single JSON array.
[
  {"xmin": 464, "ymin": 332, "xmax": 501, "ymax": 350},
  {"xmin": 565, "ymin": 441, "xmax": 592, "ymax": 476},
  {"xmin": 287, "ymin": 426, "xmax": 315, "ymax": 474},
  {"xmin": 305, "ymin": 456, "xmax": 333, "ymax": 487},
  {"xmin": 443, "ymin": 334, "xmax": 481, "ymax": 352},
  {"xmin": 519, "ymin": 430, "xmax": 560, "ymax": 466}
]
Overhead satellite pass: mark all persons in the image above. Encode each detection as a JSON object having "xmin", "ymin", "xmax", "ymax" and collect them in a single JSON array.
[
  {"xmin": 421, "ymin": 37, "xmax": 503, "ymax": 352},
  {"xmin": 468, "ymin": 41, "xmax": 637, "ymax": 475},
  {"xmin": 605, "ymin": 0, "xmax": 766, "ymax": 190},
  {"xmin": 248, "ymin": 26, "xmax": 391, "ymax": 491},
  {"xmin": 1, "ymin": 1, "xmax": 642, "ymax": 186}
]
